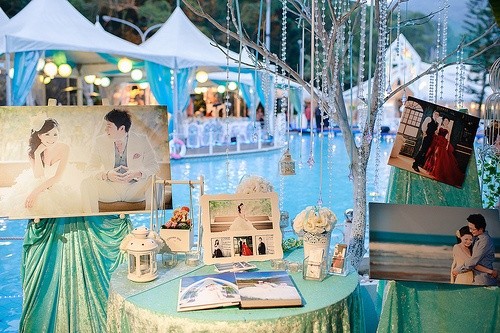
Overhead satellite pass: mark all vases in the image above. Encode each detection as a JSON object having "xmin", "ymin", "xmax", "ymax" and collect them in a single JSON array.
[{"xmin": 302, "ymin": 234, "xmax": 330, "ymax": 270}]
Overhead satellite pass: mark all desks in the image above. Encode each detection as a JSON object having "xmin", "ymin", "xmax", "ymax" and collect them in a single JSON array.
[{"xmin": 107, "ymin": 247, "xmax": 366, "ymax": 333}]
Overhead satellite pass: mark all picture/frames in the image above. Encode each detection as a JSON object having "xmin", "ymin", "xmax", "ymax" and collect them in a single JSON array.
[{"xmin": 200, "ymin": 192, "xmax": 284, "ymax": 265}]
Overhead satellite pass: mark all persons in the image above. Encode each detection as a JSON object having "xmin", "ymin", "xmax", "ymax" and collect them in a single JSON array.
[
  {"xmin": 229, "ymin": 202, "xmax": 257, "ymax": 230},
  {"xmin": 213, "ymin": 240, "xmax": 224, "ymax": 258},
  {"xmin": 258, "ymin": 237, "xmax": 266, "ymax": 255},
  {"xmin": 82, "ymin": 109, "xmax": 160, "ymax": 212},
  {"xmin": 5, "ymin": 116, "xmax": 90, "ymax": 217},
  {"xmin": 412, "ymin": 110, "xmax": 440, "ymax": 173},
  {"xmin": 424, "ymin": 116, "xmax": 455, "ymax": 180},
  {"xmin": 452, "ymin": 214, "xmax": 496, "ymax": 285},
  {"xmin": 452, "ymin": 226, "xmax": 499, "ymax": 285}
]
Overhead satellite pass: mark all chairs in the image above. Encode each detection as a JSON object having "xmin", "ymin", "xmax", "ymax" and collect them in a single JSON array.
[{"xmin": 185, "ymin": 116, "xmax": 252, "ymax": 149}]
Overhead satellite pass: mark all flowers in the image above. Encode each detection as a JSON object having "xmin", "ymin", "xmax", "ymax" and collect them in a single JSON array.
[{"xmin": 293, "ymin": 205, "xmax": 337, "ymax": 235}]
